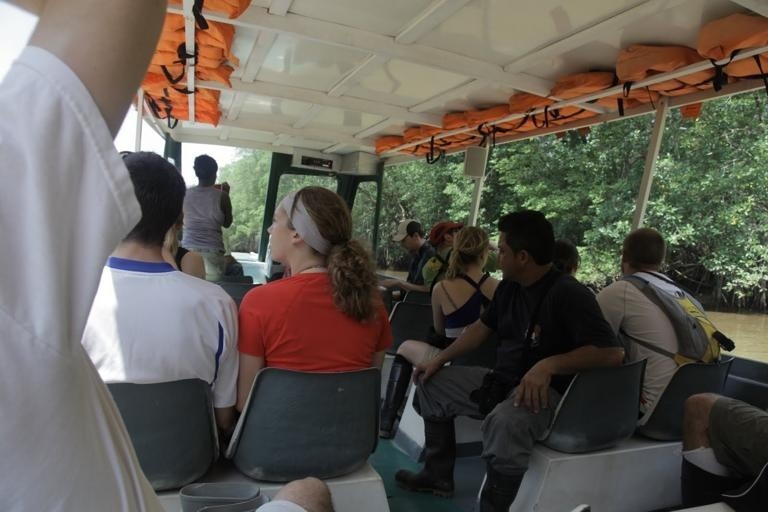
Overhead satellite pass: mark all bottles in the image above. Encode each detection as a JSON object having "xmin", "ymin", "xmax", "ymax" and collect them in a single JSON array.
[
  {"xmin": 430, "ymin": 221, "xmax": 464, "ymax": 246},
  {"xmin": 392, "ymin": 219, "xmax": 425, "ymax": 241}
]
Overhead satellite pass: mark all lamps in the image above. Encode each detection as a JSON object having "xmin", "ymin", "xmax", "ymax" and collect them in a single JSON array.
[
  {"xmin": 383, "ymin": 300, "xmax": 435, "ymax": 356},
  {"xmin": 639, "ymin": 357, "xmax": 735, "ymax": 443},
  {"xmin": 402, "ymin": 291, "xmax": 435, "ymax": 305},
  {"xmin": 220, "ymin": 283, "xmax": 263, "ymax": 309},
  {"xmin": 223, "ymin": 275, "xmax": 253, "ymax": 284},
  {"xmin": 536, "ymin": 357, "xmax": 647, "ymax": 452},
  {"xmin": 100, "ymin": 378, "xmax": 220, "ymax": 492},
  {"xmin": 224, "ymin": 367, "xmax": 380, "ymax": 482}
]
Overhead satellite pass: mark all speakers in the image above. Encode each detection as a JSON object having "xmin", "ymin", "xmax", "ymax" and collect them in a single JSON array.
[
  {"xmin": 181, "ymin": 480, "xmax": 260, "ymax": 510},
  {"xmin": 395, "ymin": 414, "xmax": 456, "ymax": 498},
  {"xmin": 379, "ymin": 355, "xmax": 413, "ymax": 438},
  {"xmin": 200, "ymin": 494, "xmax": 270, "ymax": 512},
  {"xmin": 478, "ymin": 462, "xmax": 528, "ymax": 512}
]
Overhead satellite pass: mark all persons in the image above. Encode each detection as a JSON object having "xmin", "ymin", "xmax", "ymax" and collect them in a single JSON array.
[
  {"xmin": 683, "ymin": 393, "xmax": 768, "ymax": 479},
  {"xmin": 553, "ymin": 236, "xmax": 581, "ymax": 276},
  {"xmin": 161, "ymin": 212, "xmax": 206, "ymax": 280},
  {"xmin": 379, "ymin": 219, "xmax": 437, "ymax": 315},
  {"xmin": 236, "ymin": 185, "xmax": 393, "ymax": 413},
  {"xmin": 379, "ymin": 226, "xmax": 501, "ymax": 440},
  {"xmin": 595, "ymin": 228, "xmax": 722, "ymax": 420},
  {"xmin": 80, "ymin": 151, "xmax": 239, "ymax": 439},
  {"xmin": 395, "ymin": 210, "xmax": 626, "ymax": 511},
  {"xmin": 256, "ymin": 477, "xmax": 334, "ymax": 512},
  {"xmin": 0, "ymin": 0, "xmax": 167, "ymax": 512},
  {"xmin": 422, "ymin": 221, "xmax": 498, "ymax": 285},
  {"xmin": 182, "ymin": 154, "xmax": 233, "ymax": 255}
]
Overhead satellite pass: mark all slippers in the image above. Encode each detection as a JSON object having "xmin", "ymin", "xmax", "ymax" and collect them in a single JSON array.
[{"xmin": 619, "ymin": 275, "xmax": 735, "ymax": 366}]
